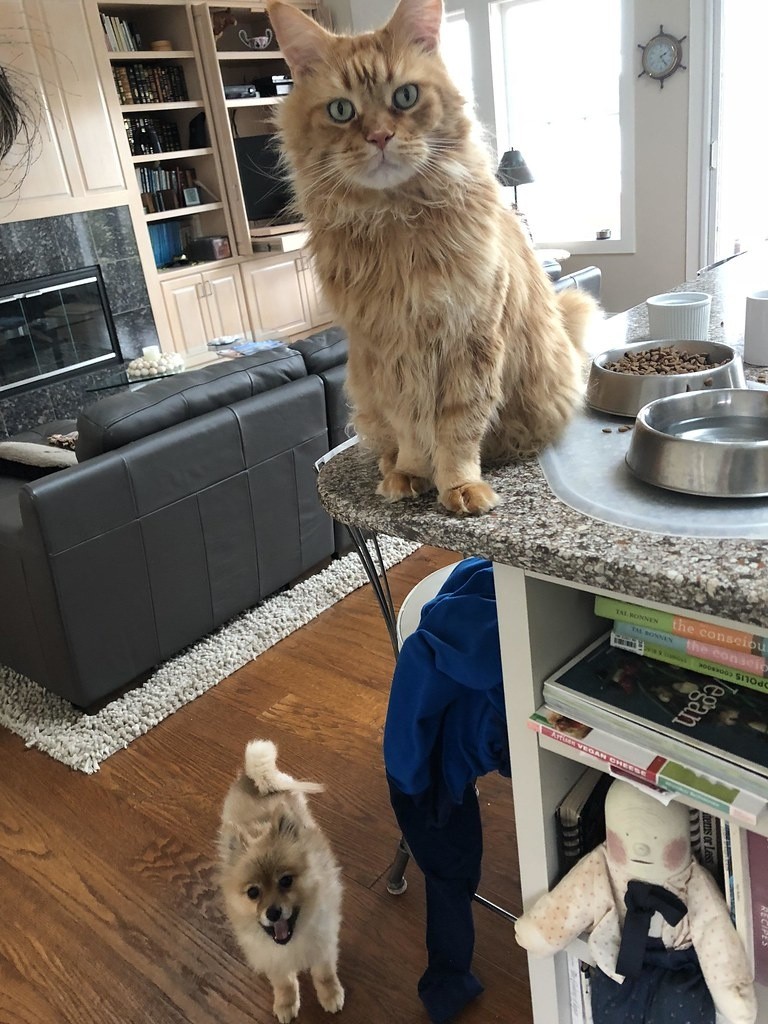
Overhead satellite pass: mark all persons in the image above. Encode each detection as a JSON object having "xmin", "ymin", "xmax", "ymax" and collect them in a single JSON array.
[{"xmin": 513, "ymin": 779, "xmax": 758, "ymax": 1024}]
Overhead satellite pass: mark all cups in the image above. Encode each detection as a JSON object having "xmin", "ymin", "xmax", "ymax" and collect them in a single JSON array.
[
  {"xmin": 646, "ymin": 291, "xmax": 712, "ymax": 342},
  {"xmin": 744, "ymin": 289, "xmax": 768, "ymax": 368},
  {"xmin": 238, "ymin": 28, "xmax": 273, "ymax": 50}
]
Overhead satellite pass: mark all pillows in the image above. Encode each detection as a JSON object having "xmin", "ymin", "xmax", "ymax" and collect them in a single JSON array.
[{"xmin": 0, "ymin": 439, "xmax": 78, "ymax": 477}]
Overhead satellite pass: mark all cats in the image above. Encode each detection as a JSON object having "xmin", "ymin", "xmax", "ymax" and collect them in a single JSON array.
[{"xmin": 262, "ymin": 2, "xmax": 599, "ymax": 518}]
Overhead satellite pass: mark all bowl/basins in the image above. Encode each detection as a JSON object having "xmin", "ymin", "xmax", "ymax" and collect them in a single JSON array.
[
  {"xmin": 584, "ymin": 339, "xmax": 749, "ymax": 418},
  {"xmin": 622, "ymin": 388, "xmax": 768, "ymax": 497}
]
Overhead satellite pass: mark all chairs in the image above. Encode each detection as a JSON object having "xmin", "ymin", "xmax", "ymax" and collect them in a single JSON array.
[{"xmin": 315, "ymin": 437, "xmax": 523, "ymax": 920}]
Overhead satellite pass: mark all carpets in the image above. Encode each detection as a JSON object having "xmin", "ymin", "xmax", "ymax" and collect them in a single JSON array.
[{"xmin": 0, "ymin": 532, "xmax": 424, "ymax": 774}]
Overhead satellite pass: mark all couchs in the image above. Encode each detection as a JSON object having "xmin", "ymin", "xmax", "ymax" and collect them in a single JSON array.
[{"xmin": 0, "ymin": 258, "xmax": 602, "ymax": 708}]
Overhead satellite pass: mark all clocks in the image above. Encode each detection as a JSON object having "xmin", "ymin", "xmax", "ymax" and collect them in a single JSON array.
[{"xmin": 637, "ymin": 24, "xmax": 687, "ymax": 91}]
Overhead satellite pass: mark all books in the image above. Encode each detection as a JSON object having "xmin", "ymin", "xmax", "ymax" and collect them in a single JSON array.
[
  {"xmin": 135, "ymin": 167, "xmax": 194, "ymax": 212},
  {"xmin": 111, "ymin": 63, "xmax": 188, "ymax": 104},
  {"xmin": 527, "ymin": 596, "xmax": 768, "ymax": 825},
  {"xmin": 100, "ymin": 13, "xmax": 137, "ymax": 52},
  {"xmin": 123, "ymin": 118, "xmax": 180, "ymax": 156},
  {"xmin": 554, "ymin": 767, "xmax": 768, "ymax": 987}
]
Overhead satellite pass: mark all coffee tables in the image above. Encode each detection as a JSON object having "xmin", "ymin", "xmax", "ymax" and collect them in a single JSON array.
[{"xmin": 85, "ymin": 334, "xmax": 292, "ymax": 391}]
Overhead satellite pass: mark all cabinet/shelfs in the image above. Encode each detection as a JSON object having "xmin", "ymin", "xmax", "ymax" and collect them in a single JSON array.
[
  {"xmin": 492, "ymin": 556, "xmax": 768, "ymax": 1024},
  {"xmin": 0, "ymin": 0, "xmax": 336, "ymax": 357}
]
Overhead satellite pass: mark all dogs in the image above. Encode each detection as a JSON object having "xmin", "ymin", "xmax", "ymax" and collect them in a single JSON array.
[{"xmin": 213, "ymin": 739, "xmax": 361, "ymax": 1024}]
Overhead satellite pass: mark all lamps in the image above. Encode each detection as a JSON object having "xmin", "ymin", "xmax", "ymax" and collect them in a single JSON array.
[{"xmin": 496, "ymin": 146, "xmax": 533, "ymax": 216}]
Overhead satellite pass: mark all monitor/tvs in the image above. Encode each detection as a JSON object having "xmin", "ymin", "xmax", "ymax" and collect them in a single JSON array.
[{"xmin": 234, "ymin": 134, "xmax": 304, "ymax": 226}]
